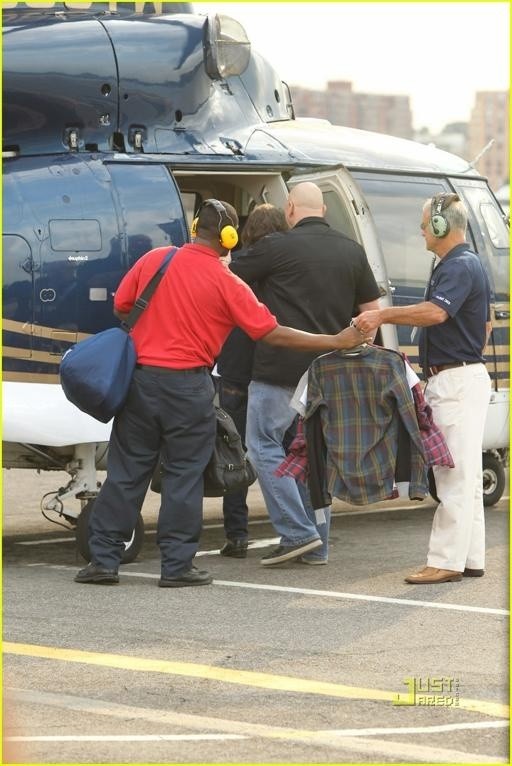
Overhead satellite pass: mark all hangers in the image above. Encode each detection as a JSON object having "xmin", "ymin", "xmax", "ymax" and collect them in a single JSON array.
[{"xmin": 341, "ymin": 319, "xmax": 370, "ymax": 351}]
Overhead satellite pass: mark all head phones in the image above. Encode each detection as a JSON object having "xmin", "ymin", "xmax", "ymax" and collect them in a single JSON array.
[
  {"xmin": 190, "ymin": 198, "xmax": 238, "ymax": 250},
  {"xmin": 429, "ymin": 193, "xmax": 460, "ymax": 238}
]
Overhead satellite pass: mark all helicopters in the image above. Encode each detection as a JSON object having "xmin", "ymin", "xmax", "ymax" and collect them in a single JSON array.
[{"xmin": 3, "ymin": 2, "xmax": 510, "ymax": 566}]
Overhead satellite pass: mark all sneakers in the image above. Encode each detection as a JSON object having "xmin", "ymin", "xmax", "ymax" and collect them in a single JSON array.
[
  {"xmin": 260, "ymin": 538, "xmax": 323, "ymax": 568},
  {"xmin": 296, "ymin": 555, "xmax": 331, "ymax": 564},
  {"xmin": 463, "ymin": 568, "xmax": 485, "ymax": 577}
]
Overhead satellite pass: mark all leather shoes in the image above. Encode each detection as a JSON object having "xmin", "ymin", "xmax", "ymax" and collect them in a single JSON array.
[
  {"xmin": 404, "ymin": 565, "xmax": 463, "ymax": 585},
  {"xmin": 73, "ymin": 560, "xmax": 121, "ymax": 587},
  {"xmin": 156, "ymin": 564, "xmax": 215, "ymax": 588},
  {"xmin": 218, "ymin": 538, "xmax": 250, "ymax": 561}
]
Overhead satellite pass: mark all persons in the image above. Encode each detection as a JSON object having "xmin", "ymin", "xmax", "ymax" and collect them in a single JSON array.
[
  {"xmin": 229, "ymin": 176, "xmax": 385, "ymax": 570},
  {"xmin": 72, "ymin": 193, "xmax": 373, "ymax": 590},
  {"xmin": 350, "ymin": 188, "xmax": 499, "ymax": 583},
  {"xmin": 218, "ymin": 200, "xmax": 290, "ymax": 559}
]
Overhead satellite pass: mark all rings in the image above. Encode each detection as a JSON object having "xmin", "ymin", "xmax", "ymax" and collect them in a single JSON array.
[{"xmin": 353, "ymin": 324, "xmax": 357, "ymax": 328}]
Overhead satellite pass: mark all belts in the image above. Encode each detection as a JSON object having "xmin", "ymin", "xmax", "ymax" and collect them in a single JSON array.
[
  {"xmin": 134, "ymin": 366, "xmax": 209, "ymax": 376},
  {"xmin": 422, "ymin": 360, "xmax": 483, "ymax": 378}
]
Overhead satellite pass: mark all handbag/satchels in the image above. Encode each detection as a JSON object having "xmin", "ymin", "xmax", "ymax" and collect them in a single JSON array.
[
  {"xmin": 150, "ymin": 405, "xmax": 258, "ymax": 499},
  {"xmin": 57, "ymin": 325, "xmax": 138, "ymax": 424}
]
